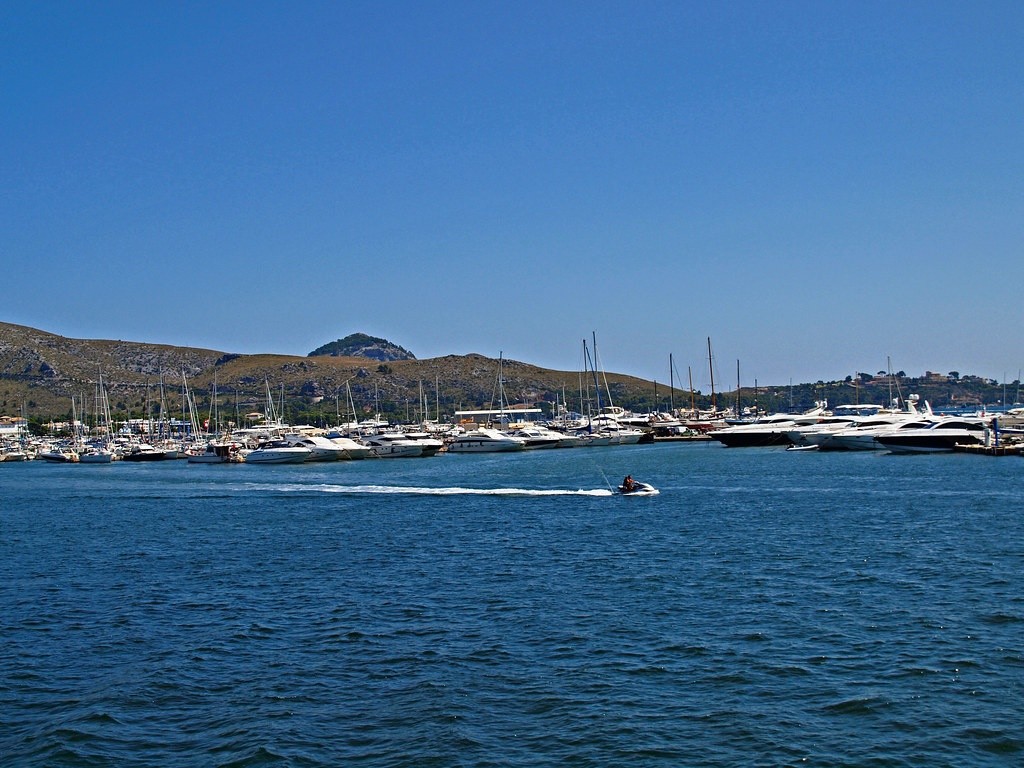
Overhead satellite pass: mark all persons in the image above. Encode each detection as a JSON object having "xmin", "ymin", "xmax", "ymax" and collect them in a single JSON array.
[
  {"xmin": 623, "ymin": 476, "xmax": 627, "ymax": 490},
  {"xmin": 627, "ymin": 474, "xmax": 633, "ymax": 490}
]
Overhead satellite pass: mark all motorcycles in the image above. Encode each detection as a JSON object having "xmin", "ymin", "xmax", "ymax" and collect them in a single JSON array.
[{"xmin": 611, "ymin": 481, "xmax": 659, "ymax": 497}]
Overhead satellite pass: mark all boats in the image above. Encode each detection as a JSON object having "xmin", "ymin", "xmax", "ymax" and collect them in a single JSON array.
[{"xmin": 0, "ymin": 333, "xmax": 1024, "ymax": 465}]
